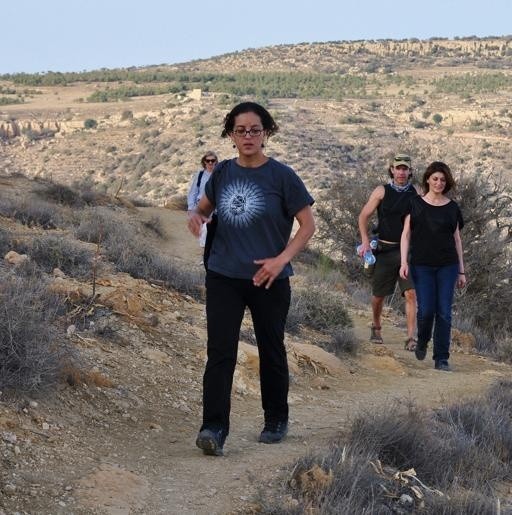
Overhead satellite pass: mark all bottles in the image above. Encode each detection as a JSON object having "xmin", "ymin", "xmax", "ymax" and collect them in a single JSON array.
[{"xmin": 355, "ymin": 243, "xmax": 376, "ymax": 265}]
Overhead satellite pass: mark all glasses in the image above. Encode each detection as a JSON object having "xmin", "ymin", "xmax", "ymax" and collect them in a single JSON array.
[
  {"xmin": 234, "ymin": 129, "xmax": 262, "ymax": 137},
  {"xmin": 205, "ymin": 159, "xmax": 216, "ymax": 162},
  {"xmin": 394, "ymin": 157, "xmax": 410, "ymax": 161}
]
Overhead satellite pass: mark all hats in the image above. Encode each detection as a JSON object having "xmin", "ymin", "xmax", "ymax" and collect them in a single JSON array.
[{"xmin": 392, "ymin": 155, "xmax": 411, "ymax": 169}]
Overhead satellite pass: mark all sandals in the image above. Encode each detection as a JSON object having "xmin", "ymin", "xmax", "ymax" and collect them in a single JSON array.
[{"xmin": 370, "ymin": 323, "xmax": 383, "ymax": 343}]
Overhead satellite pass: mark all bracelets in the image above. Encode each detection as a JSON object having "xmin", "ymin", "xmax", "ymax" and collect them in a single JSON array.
[{"xmin": 458, "ymin": 272, "xmax": 465, "ymax": 275}]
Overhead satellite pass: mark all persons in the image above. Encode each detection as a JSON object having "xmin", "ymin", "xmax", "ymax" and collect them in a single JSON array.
[
  {"xmin": 189, "ymin": 151, "xmax": 218, "ymax": 265},
  {"xmin": 356, "ymin": 153, "xmax": 421, "ymax": 351},
  {"xmin": 400, "ymin": 162, "xmax": 466, "ymax": 372},
  {"xmin": 188, "ymin": 102, "xmax": 316, "ymax": 458}
]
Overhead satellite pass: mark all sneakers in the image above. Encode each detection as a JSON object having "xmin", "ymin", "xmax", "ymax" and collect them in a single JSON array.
[
  {"xmin": 259, "ymin": 421, "xmax": 288, "ymax": 444},
  {"xmin": 435, "ymin": 359, "xmax": 449, "ymax": 370},
  {"xmin": 195, "ymin": 429, "xmax": 224, "ymax": 456},
  {"xmin": 405, "ymin": 338, "xmax": 426, "ymax": 360}
]
